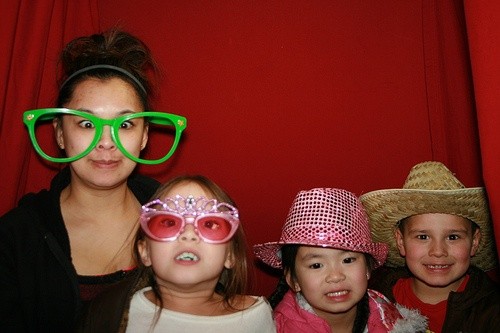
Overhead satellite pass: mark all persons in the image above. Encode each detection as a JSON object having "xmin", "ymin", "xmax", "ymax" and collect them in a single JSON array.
[
  {"xmin": 252, "ymin": 187, "xmax": 431, "ymax": 333},
  {"xmin": 1, "ymin": 26, "xmax": 180, "ymax": 332},
  {"xmin": 123, "ymin": 175, "xmax": 274, "ymax": 333},
  {"xmin": 359, "ymin": 162, "xmax": 500, "ymax": 332}
]
